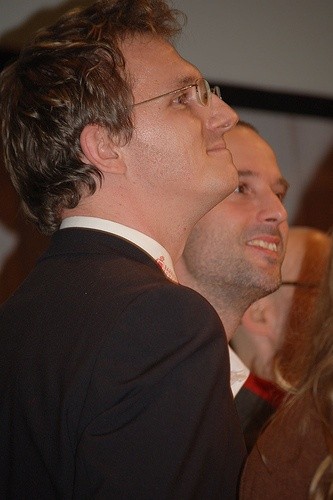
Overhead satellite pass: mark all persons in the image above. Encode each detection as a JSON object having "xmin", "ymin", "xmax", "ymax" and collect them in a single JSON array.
[
  {"xmin": 172, "ymin": 120, "xmax": 289, "ymax": 499},
  {"xmin": 0, "ymin": 0, "xmax": 241, "ymax": 499},
  {"xmin": 228, "ymin": 225, "xmax": 331, "ymax": 499}
]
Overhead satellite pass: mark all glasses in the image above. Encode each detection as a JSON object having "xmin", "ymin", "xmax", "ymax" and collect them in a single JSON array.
[{"xmin": 131, "ymin": 78, "xmax": 220, "ymax": 108}]
